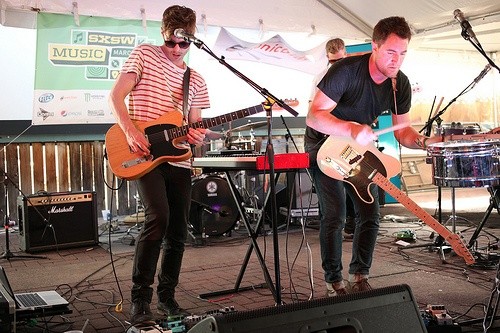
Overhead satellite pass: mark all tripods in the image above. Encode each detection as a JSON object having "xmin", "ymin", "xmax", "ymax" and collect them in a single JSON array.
[
  {"xmin": 0, "ymin": 167, "xmax": 47, "ymax": 260},
  {"xmin": 395, "ymin": 27, "xmax": 499, "ymax": 263}
]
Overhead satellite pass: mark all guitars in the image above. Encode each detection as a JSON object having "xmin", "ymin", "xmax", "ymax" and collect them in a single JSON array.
[
  {"xmin": 315, "ymin": 121, "xmax": 476, "ymax": 266},
  {"xmin": 104, "ymin": 97, "xmax": 300, "ymax": 180}
]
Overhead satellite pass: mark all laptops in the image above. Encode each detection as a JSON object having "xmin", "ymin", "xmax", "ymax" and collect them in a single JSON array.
[{"xmin": 0, "ymin": 265, "xmax": 69, "ymax": 312}]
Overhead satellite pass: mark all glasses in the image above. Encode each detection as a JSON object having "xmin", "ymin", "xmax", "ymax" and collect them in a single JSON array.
[
  {"xmin": 329, "ymin": 58, "xmax": 343, "ymax": 64},
  {"xmin": 164, "ymin": 39, "xmax": 190, "ymax": 50}
]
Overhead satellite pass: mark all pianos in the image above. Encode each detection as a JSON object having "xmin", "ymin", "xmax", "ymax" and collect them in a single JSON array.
[{"xmin": 190, "ymin": 150, "xmax": 310, "ymax": 308}]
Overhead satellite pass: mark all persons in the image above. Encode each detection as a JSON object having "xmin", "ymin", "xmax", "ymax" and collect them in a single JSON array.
[
  {"xmin": 110, "ymin": 5, "xmax": 211, "ymax": 323},
  {"xmin": 308, "ymin": 37, "xmax": 356, "ymax": 241},
  {"xmin": 194, "ymin": 129, "xmax": 225, "ymax": 149},
  {"xmin": 304, "ymin": 17, "xmax": 438, "ymax": 293}
]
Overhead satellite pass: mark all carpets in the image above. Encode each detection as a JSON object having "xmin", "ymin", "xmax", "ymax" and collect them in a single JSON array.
[{"xmin": 98, "ymin": 226, "xmax": 242, "ymax": 255}]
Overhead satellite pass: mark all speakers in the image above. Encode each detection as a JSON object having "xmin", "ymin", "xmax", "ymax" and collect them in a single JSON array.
[
  {"xmin": 287, "ymin": 170, "xmax": 320, "ymax": 208},
  {"xmin": 185, "ymin": 282, "xmax": 427, "ymax": 333},
  {"xmin": 15, "ymin": 191, "xmax": 98, "ymax": 253}
]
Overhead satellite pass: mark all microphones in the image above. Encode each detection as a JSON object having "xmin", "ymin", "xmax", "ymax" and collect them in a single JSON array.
[
  {"xmin": 218, "ymin": 211, "xmax": 230, "ymax": 217},
  {"xmin": 46, "ymin": 224, "xmax": 53, "ymax": 227},
  {"xmin": 312, "ymin": 187, "xmax": 316, "ymax": 193},
  {"xmin": 453, "ymin": 8, "xmax": 482, "ymax": 48},
  {"xmin": 174, "ymin": 28, "xmax": 204, "ymax": 44}
]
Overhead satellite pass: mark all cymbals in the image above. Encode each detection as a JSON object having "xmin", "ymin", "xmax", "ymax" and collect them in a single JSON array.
[{"xmin": 228, "ymin": 121, "xmax": 268, "ymax": 132}]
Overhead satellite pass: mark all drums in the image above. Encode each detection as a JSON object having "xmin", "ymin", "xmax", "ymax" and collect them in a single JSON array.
[
  {"xmin": 227, "ymin": 139, "xmax": 256, "ymax": 151},
  {"xmin": 427, "ymin": 138, "xmax": 500, "ymax": 189},
  {"xmin": 432, "ymin": 122, "xmax": 482, "ymax": 137},
  {"xmin": 186, "ymin": 173, "xmax": 242, "ymax": 237}
]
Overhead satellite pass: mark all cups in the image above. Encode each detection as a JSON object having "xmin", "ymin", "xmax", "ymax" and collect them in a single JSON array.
[{"xmin": 102, "ymin": 209, "xmax": 111, "ymax": 220}]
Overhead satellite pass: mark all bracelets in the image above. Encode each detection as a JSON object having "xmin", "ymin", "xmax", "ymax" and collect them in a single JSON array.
[{"xmin": 423, "ymin": 138, "xmax": 428, "ymax": 150}]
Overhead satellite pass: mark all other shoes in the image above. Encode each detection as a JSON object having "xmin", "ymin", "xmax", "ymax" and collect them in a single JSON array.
[
  {"xmin": 345, "ymin": 280, "xmax": 373, "ymax": 294},
  {"xmin": 325, "ymin": 287, "xmax": 348, "ymax": 298},
  {"xmin": 156, "ymin": 297, "xmax": 191, "ymax": 318},
  {"xmin": 128, "ymin": 297, "xmax": 154, "ymax": 324}
]
